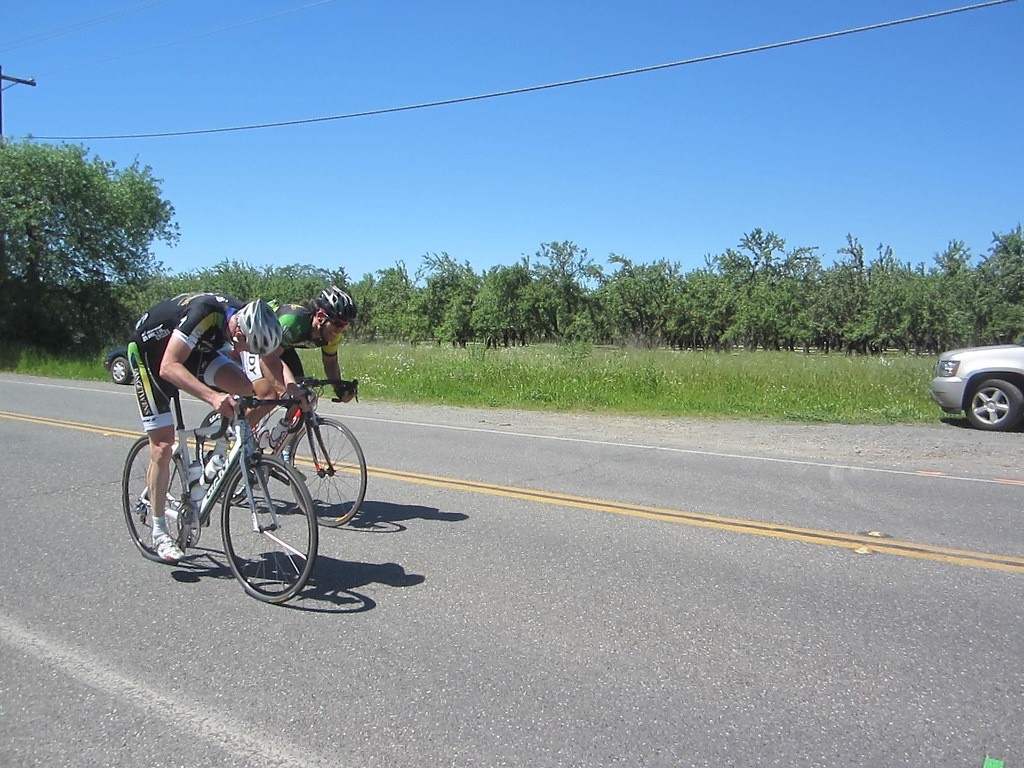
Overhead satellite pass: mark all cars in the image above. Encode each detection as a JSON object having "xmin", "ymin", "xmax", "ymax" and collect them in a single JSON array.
[
  {"xmin": 103, "ymin": 345, "xmax": 133, "ymax": 384},
  {"xmin": 928, "ymin": 338, "xmax": 1024, "ymax": 433}
]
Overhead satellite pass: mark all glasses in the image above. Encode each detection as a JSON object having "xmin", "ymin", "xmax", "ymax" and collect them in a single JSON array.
[{"xmin": 321, "ymin": 311, "xmax": 350, "ymax": 330}]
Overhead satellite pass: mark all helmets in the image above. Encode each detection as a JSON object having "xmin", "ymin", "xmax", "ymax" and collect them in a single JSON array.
[
  {"xmin": 315, "ymin": 284, "xmax": 356, "ymax": 321},
  {"xmin": 237, "ymin": 297, "xmax": 282, "ymax": 359}
]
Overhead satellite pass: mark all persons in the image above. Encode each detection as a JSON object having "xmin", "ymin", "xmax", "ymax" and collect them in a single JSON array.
[
  {"xmin": 127, "ymin": 289, "xmax": 282, "ymax": 562},
  {"xmin": 220, "ymin": 285, "xmax": 358, "ymax": 440}
]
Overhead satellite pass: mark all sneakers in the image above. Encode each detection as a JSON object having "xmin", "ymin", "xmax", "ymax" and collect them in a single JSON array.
[
  {"xmin": 151, "ymin": 531, "xmax": 184, "ymax": 561},
  {"xmin": 203, "ymin": 453, "xmax": 254, "ymax": 484}
]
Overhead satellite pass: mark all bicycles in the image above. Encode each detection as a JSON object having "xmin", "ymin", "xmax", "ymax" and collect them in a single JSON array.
[
  {"xmin": 194, "ymin": 376, "xmax": 368, "ymax": 528},
  {"xmin": 120, "ymin": 389, "xmax": 322, "ymax": 605}
]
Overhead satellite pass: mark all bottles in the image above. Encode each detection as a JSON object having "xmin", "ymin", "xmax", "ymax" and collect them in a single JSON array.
[
  {"xmin": 198, "ymin": 454, "xmax": 225, "ymax": 490},
  {"xmin": 270, "ymin": 417, "xmax": 290, "ymax": 448}
]
